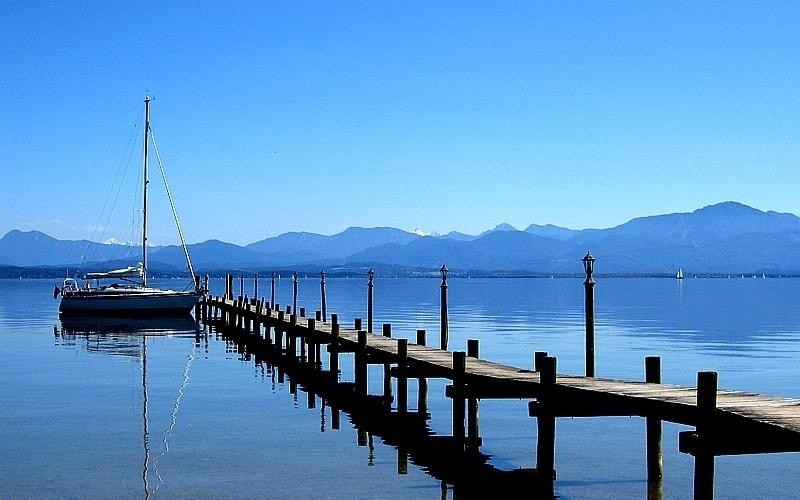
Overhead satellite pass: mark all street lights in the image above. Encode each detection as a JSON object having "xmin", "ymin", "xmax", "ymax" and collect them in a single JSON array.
[
  {"xmin": 438, "ymin": 264, "xmax": 450, "ymax": 350},
  {"xmin": 367, "ymin": 268, "xmax": 375, "ymax": 333},
  {"xmin": 239, "ymin": 270, "xmax": 326, "ymax": 323},
  {"xmin": 579, "ymin": 250, "xmax": 597, "ymax": 377}
]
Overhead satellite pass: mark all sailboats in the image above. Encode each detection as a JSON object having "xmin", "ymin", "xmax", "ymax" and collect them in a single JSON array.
[
  {"xmin": 53, "ymin": 314, "xmax": 203, "ymax": 500},
  {"xmin": 676, "ymin": 267, "xmax": 684, "ymax": 279},
  {"xmin": 60, "ymin": 93, "xmax": 208, "ymax": 316}
]
